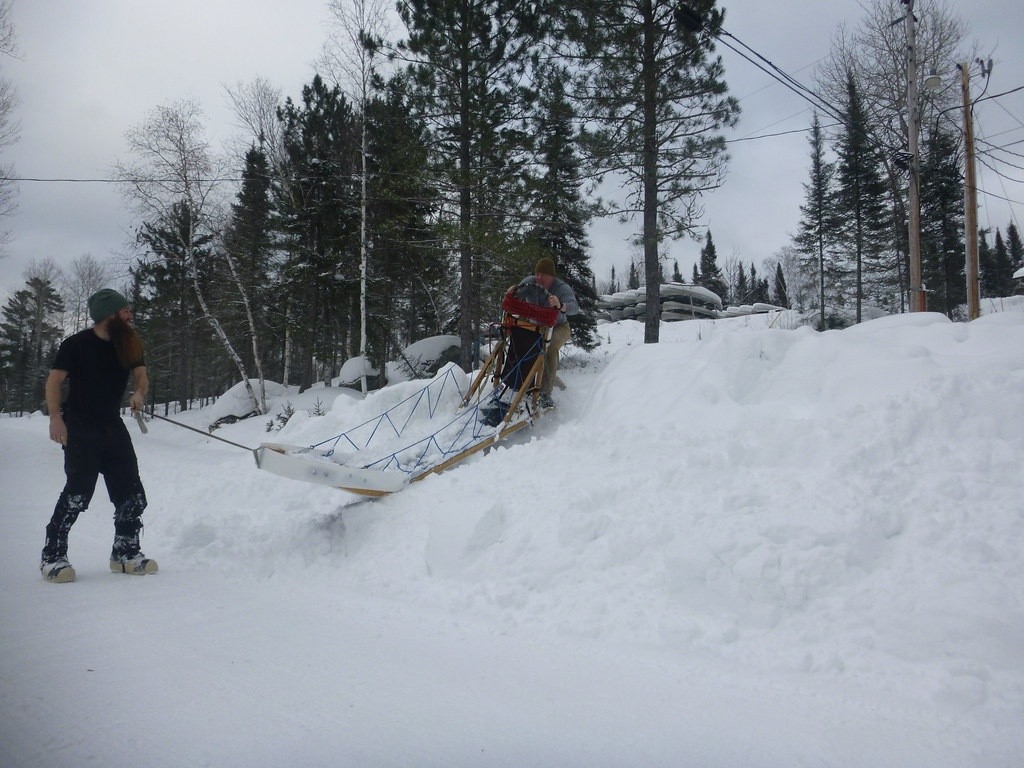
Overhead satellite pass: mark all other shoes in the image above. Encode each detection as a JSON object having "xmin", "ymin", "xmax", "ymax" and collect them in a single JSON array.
[{"xmin": 539, "ymin": 394, "xmax": 554, "ymax": 409}]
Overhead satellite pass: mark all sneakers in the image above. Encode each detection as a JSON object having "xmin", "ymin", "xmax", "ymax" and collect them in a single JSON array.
[
  {"xmin": 40, "ymin": 557, "xmax": 76, "ymax": 583},
  {"xmin": 110, "ymin": 548, "xmax": 159, "ymax": 576}
]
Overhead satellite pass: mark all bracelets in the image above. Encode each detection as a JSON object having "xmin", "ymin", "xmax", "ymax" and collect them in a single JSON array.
[{"xmin": 558, "ymin": 302, "xmax": 564, "ymax": 310}]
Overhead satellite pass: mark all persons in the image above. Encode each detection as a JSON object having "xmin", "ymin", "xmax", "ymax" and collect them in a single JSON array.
[
  {"xmin": 39, "ymin": 288, "xmax": 159, "ymax": 583},
  {"xmin": 502, "ymin": 257, "xmax": 579, "ymax": 415}
]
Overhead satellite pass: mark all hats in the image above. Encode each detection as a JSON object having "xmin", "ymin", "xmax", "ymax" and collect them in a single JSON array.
[
  {"xmin": 87, "ymin": 288, "xmax": 128, "ymax": 323},
  {"xmin": 535, "ymin": 257, "xmax": 556, "ymax": 276}
]
